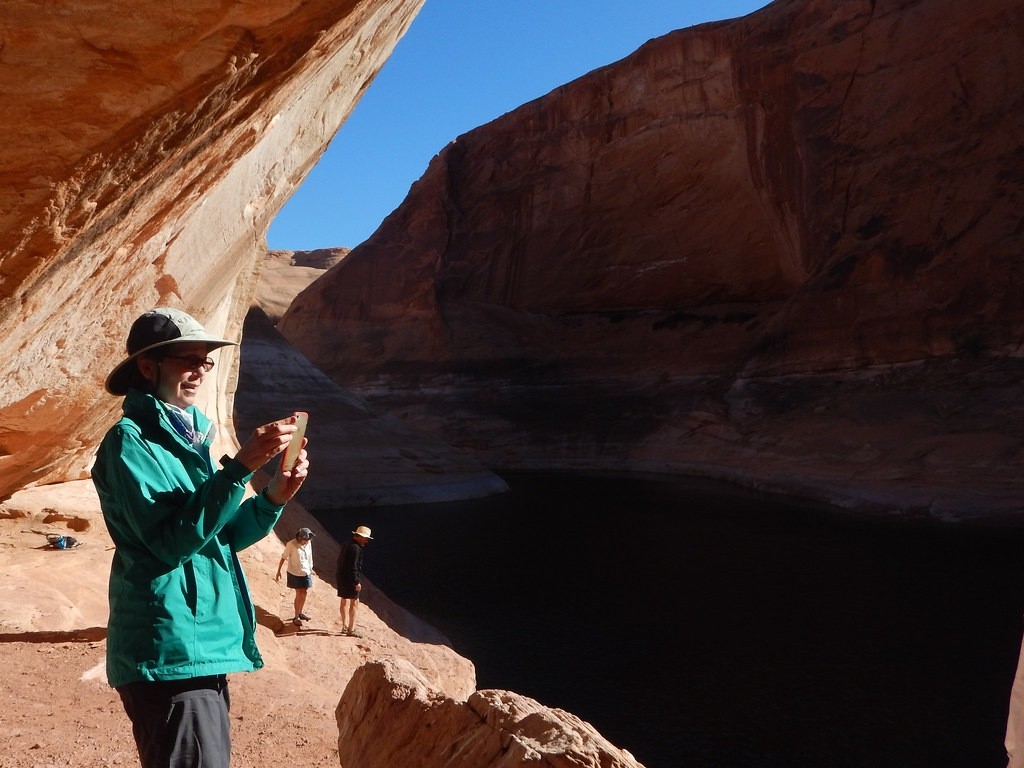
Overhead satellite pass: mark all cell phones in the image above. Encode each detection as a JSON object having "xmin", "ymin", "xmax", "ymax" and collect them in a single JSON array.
[{"xmin": 281, "ymin": 412, "xmax": 308, "ymax": 472}]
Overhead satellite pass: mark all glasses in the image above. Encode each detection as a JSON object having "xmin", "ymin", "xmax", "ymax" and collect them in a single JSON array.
[{"xmin": 165, "ymin": 354, "xmax": 214, "ymax": 372}]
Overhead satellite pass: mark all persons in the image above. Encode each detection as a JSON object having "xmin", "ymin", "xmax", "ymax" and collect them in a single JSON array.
[
  {"xmin": 337, "ymin": 526, "xmax": 374, "ymax": 637},
  {"xmin": 276, "ymin": 527, "xmax": 316, "ymax": 626},
  {"xmin": 89, "ymin": 307, "xmax": 310, "ymax": 768}
]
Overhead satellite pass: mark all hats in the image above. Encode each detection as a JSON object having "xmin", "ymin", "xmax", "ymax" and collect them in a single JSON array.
[
  {"xmin": 299, "ymin": 528, "xmax": 316, "ymax": 539},
  {"xmin": 351, "ymin": 526, "xmax": 374, "ymax": 540},
  {"xmin": 105, "ymin": 307, "xmax": 239, "ymax": 397}
]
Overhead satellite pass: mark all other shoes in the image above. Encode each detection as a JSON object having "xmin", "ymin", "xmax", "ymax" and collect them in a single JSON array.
[
  {"xmin": 347, "ymin": 628, "xmax": 363, "ymax": 638},
  {"xmin": 298, "ymin": 614, "xmax": 311, "ymax": 620},
  {"xmin": 293, "ymin": 618, "xmax": 303, "ymax": 626},
  {"xmin": 342, "ymin": 626, "xmax": 356, "ymax": 634}
]
